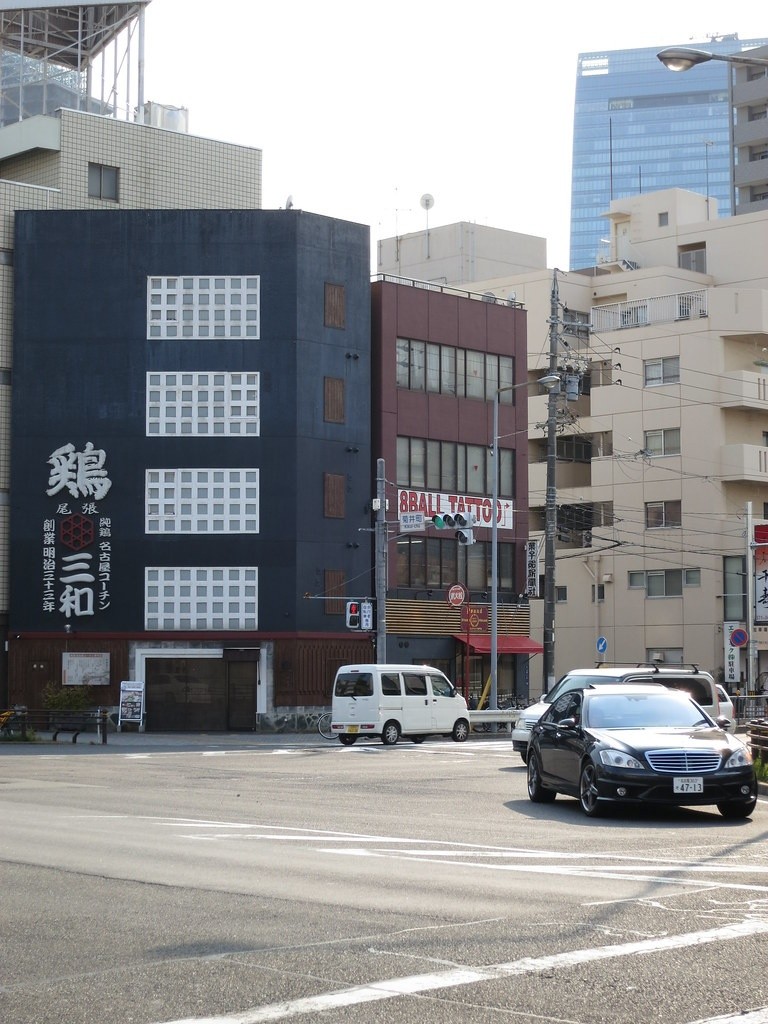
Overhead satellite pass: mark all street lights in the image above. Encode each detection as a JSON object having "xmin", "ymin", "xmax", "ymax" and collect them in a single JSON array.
[{"xmin": 490, "ymin": 374, "xmax": 562, "ymax": 731}]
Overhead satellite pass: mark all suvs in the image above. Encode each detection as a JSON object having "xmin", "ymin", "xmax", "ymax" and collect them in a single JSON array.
[{"xmin": 510, "ymin": 661, "xmax": 720, "ymax": 764}]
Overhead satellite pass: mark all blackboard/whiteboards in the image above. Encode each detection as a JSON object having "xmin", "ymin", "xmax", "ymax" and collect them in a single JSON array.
[{"xmin": 119, "ymin": 680, "xmax": 145, "ymax": 720}]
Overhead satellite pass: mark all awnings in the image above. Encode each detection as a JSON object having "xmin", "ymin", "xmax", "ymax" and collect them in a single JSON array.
[{"xmin": 452, "ymin": 634, "xmax": 545, "ymax": 654}]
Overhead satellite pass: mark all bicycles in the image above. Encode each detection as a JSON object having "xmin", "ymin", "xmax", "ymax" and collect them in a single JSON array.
[
  {"xmin": 0, "ymin": 704, "xmax": 34, "ymax": 741},
  {"xmin": 471, "ymin": 692, "xmax": 525, "ymax": 735},
  {"xmin": 318, "ymin": 711, "xmax": 340, "ymax": 739}
]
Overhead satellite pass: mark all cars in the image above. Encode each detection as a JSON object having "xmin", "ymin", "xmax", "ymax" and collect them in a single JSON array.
[
  {"xmin": 714, "ymin": 683, "xmax": 737, "ymax": 736},
  {"xmin": 525, "ymin": 684, "xmax": 759, "ymax": 820}
]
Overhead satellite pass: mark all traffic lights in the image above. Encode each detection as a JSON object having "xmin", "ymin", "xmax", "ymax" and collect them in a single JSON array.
[
  {"xmin": 431, "ymin": 512, "xmax": 473, "ymax": 531},
  {"xmin": 345, "ymin": 601, "xmax": 362, "ymax": 631},
  {"xmin": 454, "ymin": 530, "xmax": 474, "ymax": 547}
]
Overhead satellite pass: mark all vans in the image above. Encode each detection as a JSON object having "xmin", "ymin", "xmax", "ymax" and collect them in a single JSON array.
[{"xmin": 331, "ymin": 665, "xmax": 470, "ymax": 744}]
